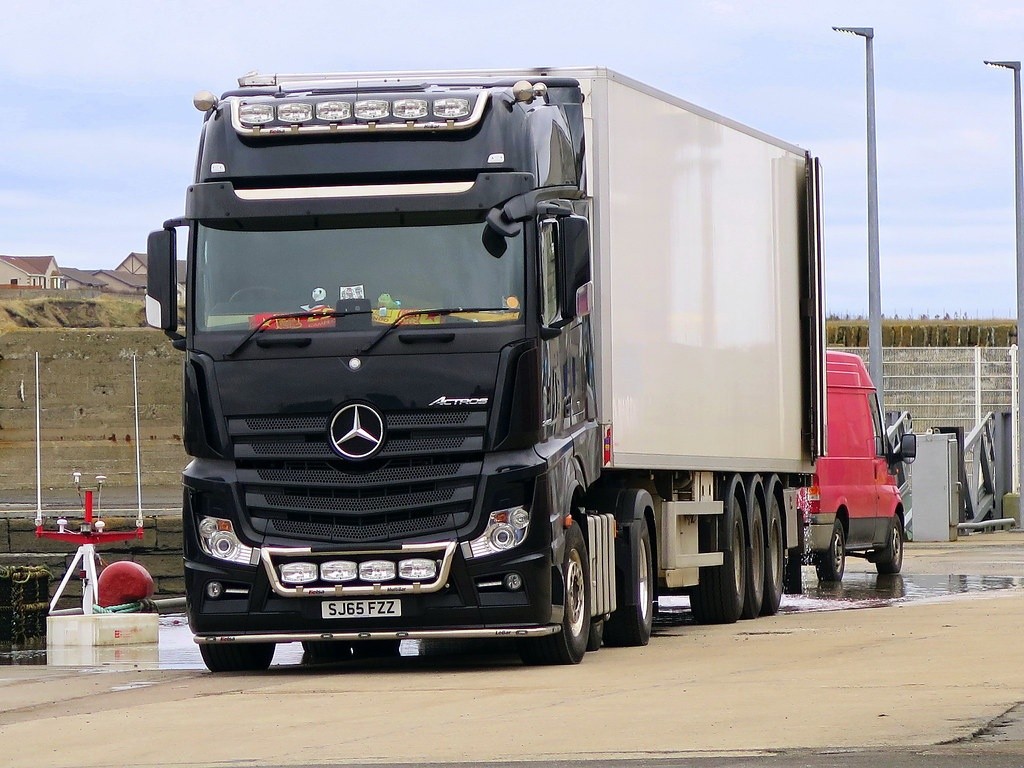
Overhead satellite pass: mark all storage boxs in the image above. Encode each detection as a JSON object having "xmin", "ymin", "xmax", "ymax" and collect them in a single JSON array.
[{"xmin": 46, "ymin": 613, "xmax": 160, "ymax": 645}]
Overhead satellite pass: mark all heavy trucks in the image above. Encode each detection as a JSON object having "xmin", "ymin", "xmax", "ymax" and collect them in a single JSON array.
[{"xmin": 145, "ymin": 64, "xmax": 827, "ymax": 670}]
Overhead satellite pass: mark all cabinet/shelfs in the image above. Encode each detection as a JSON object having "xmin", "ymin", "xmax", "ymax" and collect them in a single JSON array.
[{"xmin": 912, "ymin": 428, "xmax": 961, "ymax": 542}]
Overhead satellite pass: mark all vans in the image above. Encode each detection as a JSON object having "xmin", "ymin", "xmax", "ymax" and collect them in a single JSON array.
[{"xmin": 785, "ymin": 351, "xmax": 916, "ymax": 593}]
